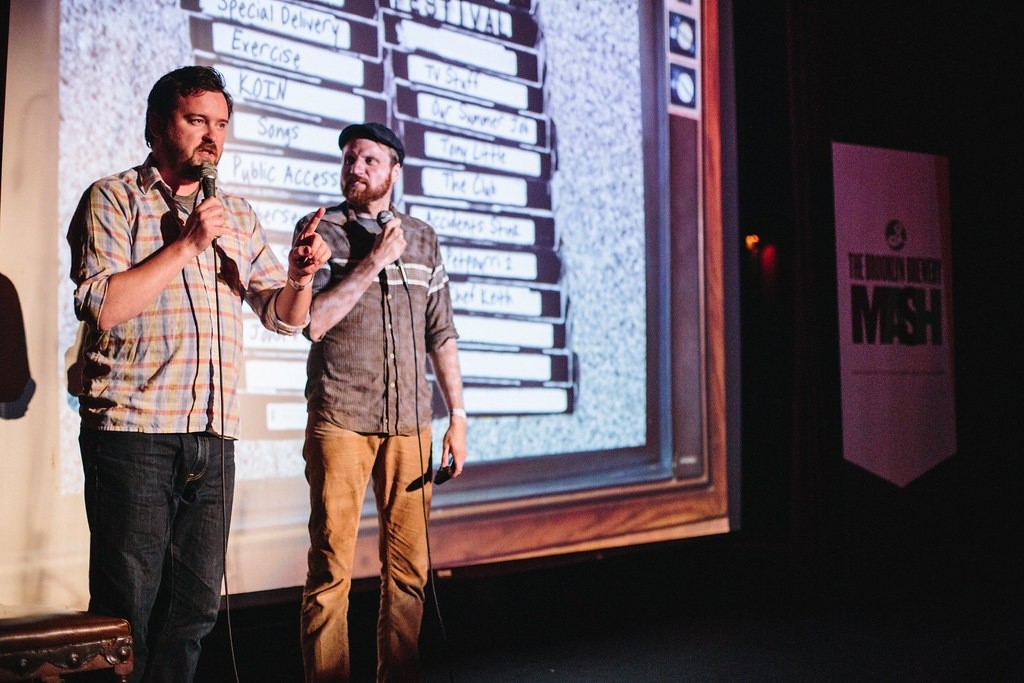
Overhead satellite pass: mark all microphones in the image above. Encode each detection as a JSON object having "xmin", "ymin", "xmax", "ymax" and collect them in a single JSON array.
[
  {"xmin": 200, "ymin": 161, "xmax": 218, "ymax": 247},
  {"xmin": 377, "ymin": 211, "xmax": 408, "ymax": 290}
]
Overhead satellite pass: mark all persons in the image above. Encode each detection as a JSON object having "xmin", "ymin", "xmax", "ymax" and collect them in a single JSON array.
[
  {"xmin": 66, "ymin": 65, "xmax": 333, "ymax": 683},
  {"xmin": 292, "ymin": 121, "xmax": 469, "ymax": 683}
]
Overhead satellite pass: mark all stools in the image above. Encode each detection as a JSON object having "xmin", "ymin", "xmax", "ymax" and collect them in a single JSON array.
[{"xmin": 0, "ymin": 612, "xmax": 134, "ymax": 683}]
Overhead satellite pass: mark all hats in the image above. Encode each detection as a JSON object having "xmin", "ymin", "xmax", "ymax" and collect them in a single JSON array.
[{"xmin": 338, "ymin": 122, "xmax": 404, "ymax": 167}]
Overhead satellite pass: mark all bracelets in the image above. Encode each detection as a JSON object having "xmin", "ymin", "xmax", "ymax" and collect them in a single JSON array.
[
  {"xmin": 288, "ymin": 274, "xmax": 314, "ymax": 291},
  {"xmin": 450, "ymin": 408, "xmax": 468, "ymax": 418}
]
